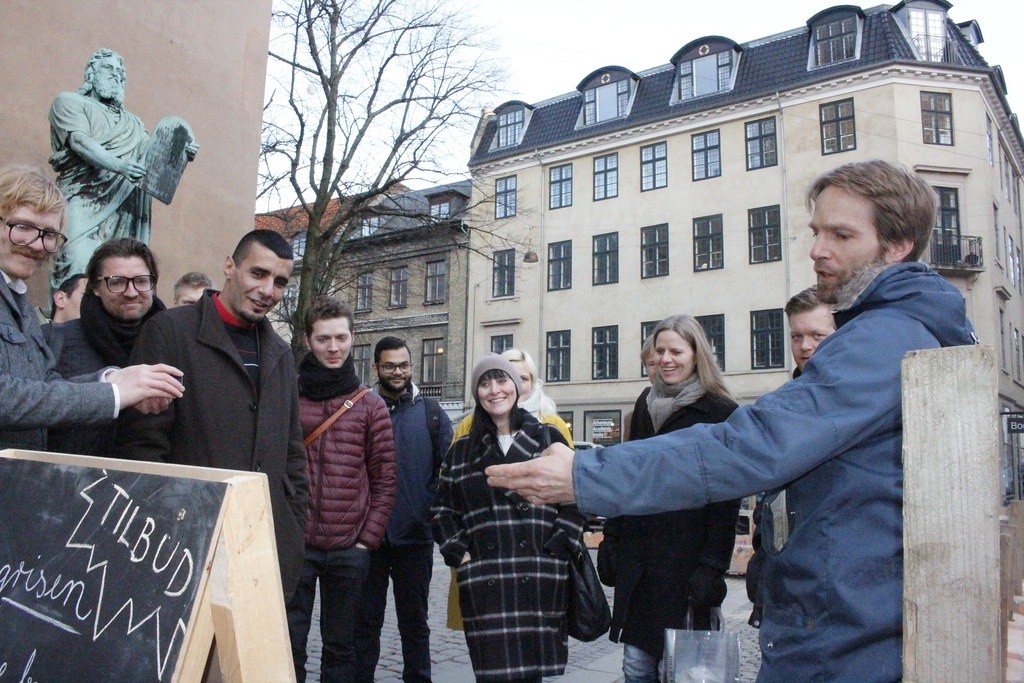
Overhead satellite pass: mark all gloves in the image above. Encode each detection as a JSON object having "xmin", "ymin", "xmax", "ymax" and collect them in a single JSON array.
[
  {"xmin": 597, "ymin": 535, "xmax": 615, "ymax": 587},
  {"xmin": 682, "ymin": 564, "xmax": 728, "ymax": 607}
]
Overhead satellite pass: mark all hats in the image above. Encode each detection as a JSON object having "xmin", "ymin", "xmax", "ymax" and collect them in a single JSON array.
[{"xmin": 471, "ymin": 352, "xmax": 523, "ymax": 399}]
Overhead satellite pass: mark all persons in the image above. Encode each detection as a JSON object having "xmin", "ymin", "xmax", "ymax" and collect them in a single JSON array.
[
  {"xmin": 371, "ymin": 336, "xmax": 453, "ymax": 683},
  {"xmin": 446, "ymin": 350, "xmax": 574, "ymax": 629},
  {"xmin": 49, "ymin": 48, "xmax": 199, "ymax": 301},
  {"xmin": 0, "ymin": 166, "xmax": 185, "ymax": 451},
  {"xmin": 36, "ymin": 273, "xmax": 88, "ymax": 324},
  {"xmin": 173, "ymin": 272, "xmax": 213, "ymax": 306},
  {"xmin": 596, "ymin": 315, "xmax": 741, "ymax": 682},
  {"xmin": 431, "ymin": 353, "xmax": 587, "ymax": 683},
  {"xmin": 125, "ymin": 229, "xmax": 308, "ymax": 610},
  {"xmin": 746, "ymin": 286, "xmax": 837, "ymax": 628},
  {"xmin": 623, "ymin": 336, "xmax": 655, "ymax": 443},
  {"xmin": 295, "ymin": 301, "xmax": 397, "ymax": 683},
  {"xmin": 485, "ymin": 160, "xmax": 980, "ymax": 683},
  {"xmin": 39, "ymin": 239, "xmax": 167, "ymax": 455}
]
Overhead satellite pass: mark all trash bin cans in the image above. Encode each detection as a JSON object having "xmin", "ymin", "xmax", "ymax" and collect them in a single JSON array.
[{"xmin": 725, "ymin": 510, "xmax": 754, "ymax": 575}]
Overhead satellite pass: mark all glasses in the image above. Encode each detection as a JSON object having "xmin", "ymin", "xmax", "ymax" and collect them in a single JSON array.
[
  {"xmin": 0, "ymin": 216, "xmax": 68, "ymax": 254},
  {"xmin": 96, "ymin": 272, "xmax": 157, "ymax": 294},
  {"xmin": 378, "ymin": 362, "xmax": 413, "ymax": 374}
]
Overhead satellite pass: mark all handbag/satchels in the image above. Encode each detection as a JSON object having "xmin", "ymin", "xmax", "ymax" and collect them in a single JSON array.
[
  {"xmin": 663, "ymin": 606, "xmax": 739, "ymax": 683},
  {"xmin": 566, "ymin": 539, "xmax": 612, "ymax": 642}
]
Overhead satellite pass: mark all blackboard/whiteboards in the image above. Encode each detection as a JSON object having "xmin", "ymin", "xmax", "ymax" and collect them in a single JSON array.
[{"xmin": 0, "ymin": 449, "xmax": 297, "ymax": 682}]
